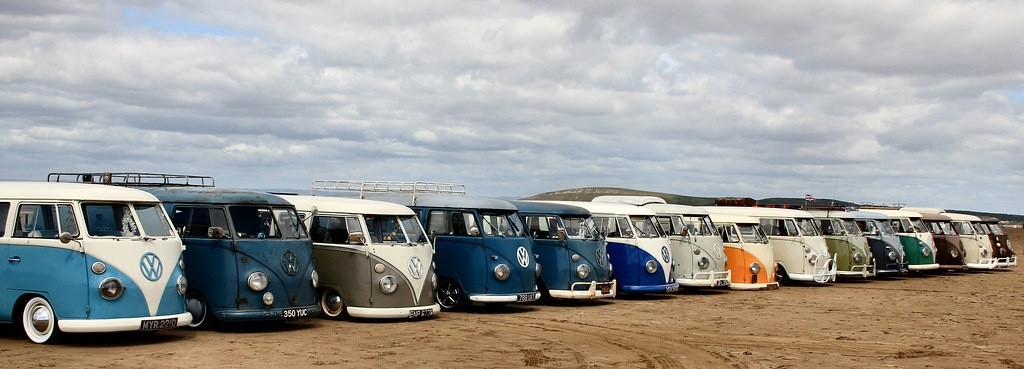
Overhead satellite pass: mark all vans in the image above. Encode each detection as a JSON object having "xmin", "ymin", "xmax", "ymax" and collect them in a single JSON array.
[
  {"xmin": 590, "ymin": 194, "xmax": 732, "ymax": 290},
  {"xmin": 0, "ymin": 180, "xmax": 194, "ymax": 346},
  {"xmin": 852, "ymin": 209, "xmax": 941, "ymax": 273},
  {"xmin": 518, "ymin": 198, "xmax": 680, "ymax": 297},
  {"xmin": 652, "ymin": 215, "xmax": 780, "ymax": 292},
  {"xmin": 805, "ymin": 207, "xmax": 908, "ymax": 277},
  {"xmin": 889, "ymin": 206, "xmax": 1018, "ymax": 273},
  {"xmin": 688, "ymin": 206, "xmax": 838, "ymax": 284},
  {"xmin": 45, "ymin": 170, "xmax": 321, "ymax": 330},
  {"xmin": 465, "ymin": 198, "xmax": 618, "ymax": 304},
  {"xmin": 256, "ymin": 192, "xmax": 443, "ymax": 320},
  {"xmin": 304, "ymin": 178, "xmax": 544, "ymax": 309},
  {"xmin": 814, "ymin": 215, "xmax": 877, "ymax": 279}
]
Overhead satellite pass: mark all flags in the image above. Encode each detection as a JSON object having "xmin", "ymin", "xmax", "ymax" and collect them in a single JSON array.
[{"xmin": 806, "ymin": 194, "xmax": 816, "ymax": 202}]
